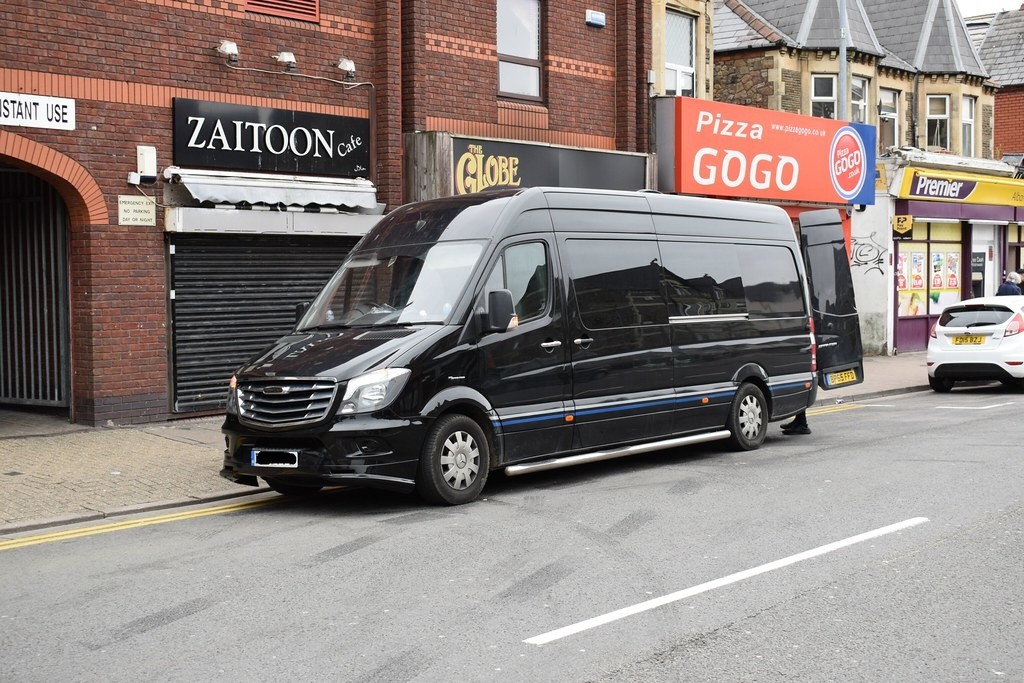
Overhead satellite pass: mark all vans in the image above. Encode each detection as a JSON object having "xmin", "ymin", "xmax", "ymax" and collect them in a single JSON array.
[{"xmin": 219, "ymin": 186, "xmax": 865, "ymax": 505}]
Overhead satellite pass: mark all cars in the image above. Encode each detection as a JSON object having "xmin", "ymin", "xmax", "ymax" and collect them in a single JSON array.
[{"xmin": 926, "ymin": 295, "xmax": 1024, "ymax": 393}]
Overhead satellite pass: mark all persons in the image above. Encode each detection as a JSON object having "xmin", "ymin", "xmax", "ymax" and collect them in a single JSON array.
[
  {"xmin": 995, "ymin": 271, "xmax": 1022, "ymax": 296},
  {"xmin": 1016, "ymin": 269, "xmax": 1024, "ymax": 295}
]
